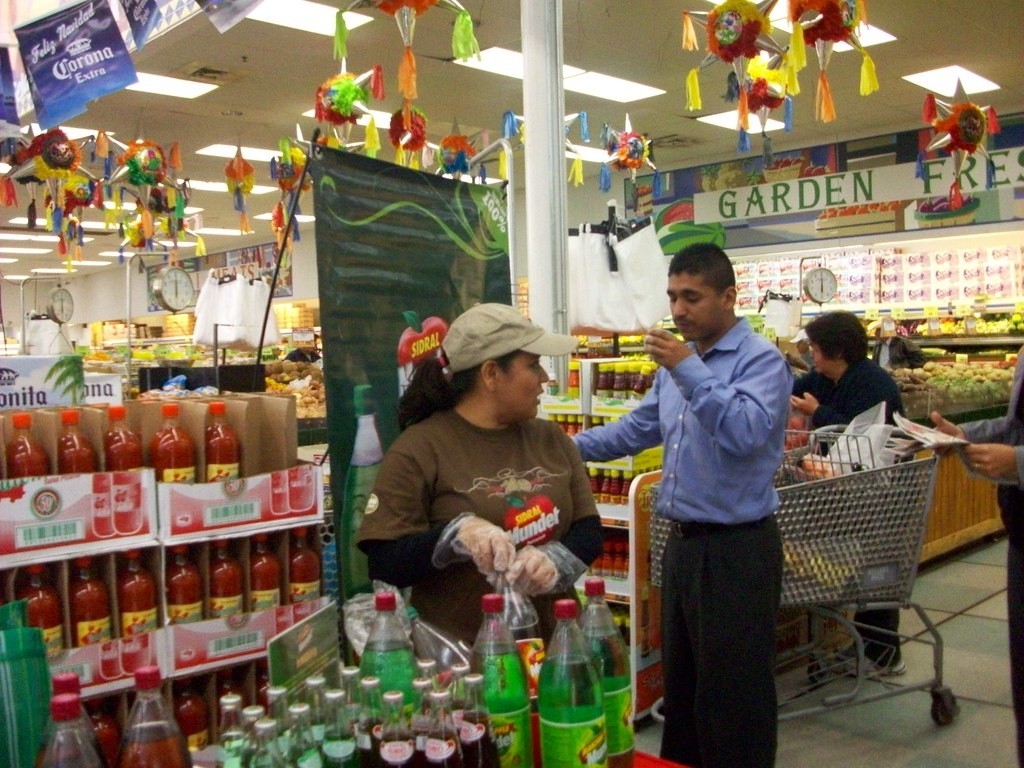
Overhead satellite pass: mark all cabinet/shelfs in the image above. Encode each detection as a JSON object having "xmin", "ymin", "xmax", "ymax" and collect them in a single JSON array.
[{"xmin": 537, "ymin": 399, "xmax": 857, "ymax": 735}]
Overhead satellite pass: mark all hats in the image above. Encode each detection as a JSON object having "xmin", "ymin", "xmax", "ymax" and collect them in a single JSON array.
[{"xmin": 441, "ymin": 301, "xmax": 579, "ymax": 375}]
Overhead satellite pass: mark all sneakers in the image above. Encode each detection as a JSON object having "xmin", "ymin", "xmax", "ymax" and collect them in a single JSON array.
[
  {"xmin": 835, "ymin": 640, "xmax": 870, "ymax": 661},
  {"xmin": 843, "ymin": 656, "xmax": 907, "ymax": 680}
]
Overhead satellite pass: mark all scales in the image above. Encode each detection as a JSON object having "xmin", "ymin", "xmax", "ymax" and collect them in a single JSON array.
[
  {"xmin": 799, "ymin": 254, "xmax": 838, "ymax": 317},
  {"xmin": 45, "ymin": 281, "xmax": 74, "ymax": 354},
  {"xmin": 153, "ymin": 253, "xmax": 195, "ymax": 369}
]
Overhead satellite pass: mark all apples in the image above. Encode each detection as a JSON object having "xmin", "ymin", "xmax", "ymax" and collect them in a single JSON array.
[{"xmin": 916, "ymin": 311, "xmax": 1024, "ymax": 336}]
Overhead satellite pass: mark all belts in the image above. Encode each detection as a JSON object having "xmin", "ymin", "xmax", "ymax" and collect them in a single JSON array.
[{"xmin": 668, "ymin": 514, "xmax": 772, "ymax": 539}]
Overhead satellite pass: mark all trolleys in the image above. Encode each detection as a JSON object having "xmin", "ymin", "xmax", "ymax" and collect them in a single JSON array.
[{"xmin": 650, "ymin": 424, "xmax": 961, "ymax": 726}]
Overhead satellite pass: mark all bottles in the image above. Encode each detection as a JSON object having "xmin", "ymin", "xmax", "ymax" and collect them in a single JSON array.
[
  {"xmin": 303, "ymin": 576, "xmax": 635, "ymax": 767},
  {"xmin": 340, "ymin": 384, "xmax": 385, "ymax": 667},
  {"xmin": 7, "ymin": 402, "xmax": 321, "ymax": 768},
  {"xmin": 546, "ymin": 361, "xmax": 661, "ymax": 579},
  {"xmin": 640, "ymin": 587, "xmax": 651, "ymax": 659}
]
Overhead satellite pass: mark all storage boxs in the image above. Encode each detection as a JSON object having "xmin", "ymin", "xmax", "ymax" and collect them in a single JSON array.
[
  {"xmin": 0, "ymin": 355, "xmax": 330, "ymax": 743},
  {"xmin": 89, "ymin": 296, "xmax": 314, "ymax": 349},
  {"xmin": 773, "ymin": 606, "xmax": 856, "ymax": 706}
]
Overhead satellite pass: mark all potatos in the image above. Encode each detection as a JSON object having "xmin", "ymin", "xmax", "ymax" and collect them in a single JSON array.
[
  {"xmin": 784, "ymin": 350, "xmax": 808, "ymax": 379},
  {"xmin": 889, "ymin": 360, "xmax": 1016, "ymax": 416},
  {"xmin": 262, "ymin": 360, "xmax": 326, "ymax": 418}
]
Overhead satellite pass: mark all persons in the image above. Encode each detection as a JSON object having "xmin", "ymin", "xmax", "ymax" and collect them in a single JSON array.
[
  {"xmin": 791, "ymin": 311, "xmax": 907, "ymax": 679},
  {"xmin": 574, "ymin": 242, "xmax": 794, "ymax": 767},
  {"xmin": 928, "ymin": 344, "xmax": 1023, "ymax": 768},
  {"xmin": 867, "ymin": 316, "xmax": 925, "ymax": 370},
  {"xmin": 286, "ymin": 334, "xmax": 321, "ymax": 363},
  {"xmin": 975, "ymin": 463, "xmax": 978, "ymax": 471},
  {"xmin": 355, "ymin": 303, "xmax": 606, "ymax": 644},
  {"xmin": 787, "ymin": 329, "xmax": 815, "ymax": 371}
]
